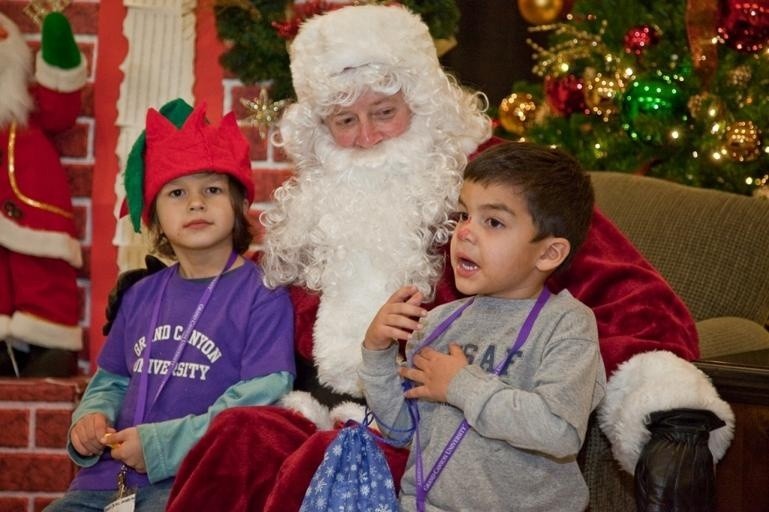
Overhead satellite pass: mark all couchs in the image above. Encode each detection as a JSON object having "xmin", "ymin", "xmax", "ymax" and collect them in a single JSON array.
[{"xmin": 579, "ymin": 169, "xmax": 765, "ymax": 512}]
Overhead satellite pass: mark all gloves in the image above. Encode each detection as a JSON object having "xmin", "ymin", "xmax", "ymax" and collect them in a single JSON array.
[
  {"xmin": 33, "ymin": 10, "xmax": 94, "ymax": 91},
  {"xmin": 631, "ymin": 408, "xmax": 725, "ymax": 511}
]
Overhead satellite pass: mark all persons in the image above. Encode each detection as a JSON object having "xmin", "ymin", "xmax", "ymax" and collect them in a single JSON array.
[
  {"xmin": 355, "ymin": 140, "xmax": 606, "ymax": 512},
  {"xmin": 0, "ymin": 0, "xmax": 92, "ymax": 382},
  {"xmin": 99, "ymin": 0, "xmax": 741, "ymax": 512},
  {"xmin": 36, "ymin": 96, "xmax": 299, "ymax": 511}
]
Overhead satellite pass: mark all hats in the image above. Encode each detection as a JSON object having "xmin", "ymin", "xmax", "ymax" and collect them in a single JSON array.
[
  {"xmin": 287, "ymin": 0, "xmax": 437, "ymax": 102},
  {"xmin": 124, "ymin": 98, "xmax": 257, "ymax": 232}
]
